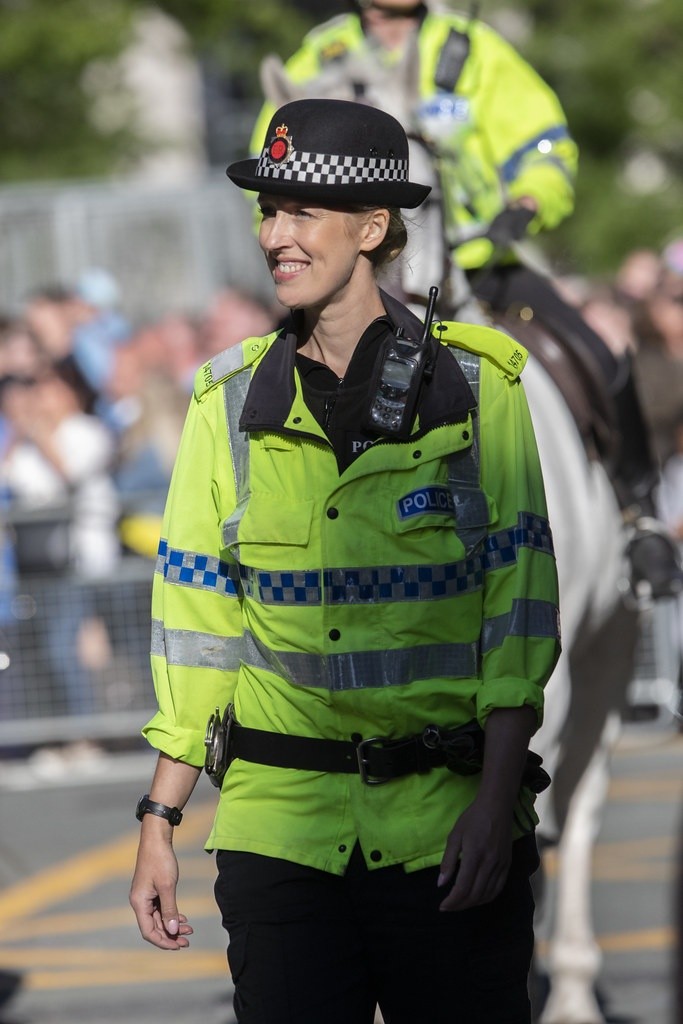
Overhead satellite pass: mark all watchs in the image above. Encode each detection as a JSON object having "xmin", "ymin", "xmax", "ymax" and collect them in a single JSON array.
[{"xmin": 132, "ymin": 796, "xmax": 184, "ymax": 829}]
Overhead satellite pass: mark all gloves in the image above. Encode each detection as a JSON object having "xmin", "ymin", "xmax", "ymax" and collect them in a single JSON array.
[{"xmin": 487, "ymin": 207, "xmax": 535, "ymax": 247}]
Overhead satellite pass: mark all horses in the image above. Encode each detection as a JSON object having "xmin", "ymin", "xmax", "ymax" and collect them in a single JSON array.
[{"xmin": 258, "ymin": 18, "xmax": 641, "ymax": 1023}]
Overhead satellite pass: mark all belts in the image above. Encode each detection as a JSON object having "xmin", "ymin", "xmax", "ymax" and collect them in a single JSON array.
[{"xmin": 228, "ymin": 721, "xmax": 485, "ymax": 784}]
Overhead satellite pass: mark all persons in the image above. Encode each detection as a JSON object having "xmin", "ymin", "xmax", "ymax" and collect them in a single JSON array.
[
  {"xmin": 128, "ymin": 98, "xmax": 561, "ymax": 1024},
  {"xmin": 242, "ymin": 0, "xmax": 681, "ymax": 609}
]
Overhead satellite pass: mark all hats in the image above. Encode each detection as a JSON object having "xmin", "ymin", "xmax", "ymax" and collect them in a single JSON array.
[{"xmin": 226, "ymin": 98, "xmax": 432, "ymax": 210}]
